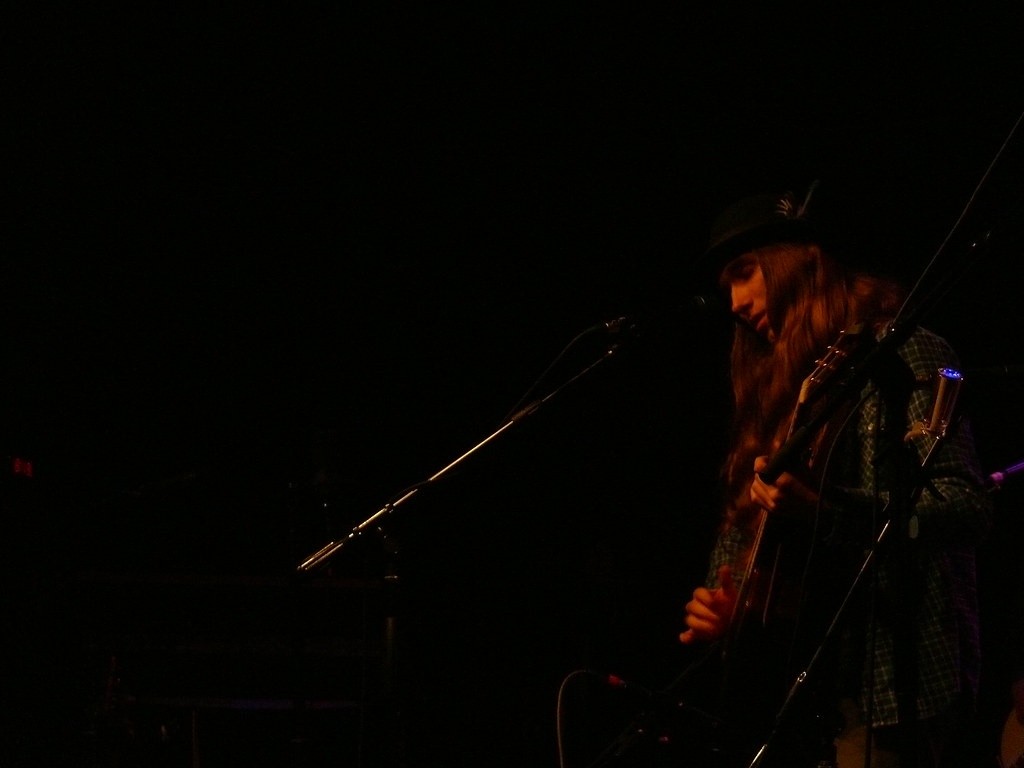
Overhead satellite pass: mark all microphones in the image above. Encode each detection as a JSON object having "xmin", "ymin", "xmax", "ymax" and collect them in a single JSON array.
[
  {"xmin": 588, "ymin": 668, "xmax": 727, "ymax": 734},
  {"xmin": 905, "ymin": 364, "xmax": 1024, "ymax": 386},
  {"xmin": 605, "ymin": 297, "xmax": 707, "ymax": 336},
  {"xmin": 929, "ymin": 367, "xmax": 963, "ymax": 439}
]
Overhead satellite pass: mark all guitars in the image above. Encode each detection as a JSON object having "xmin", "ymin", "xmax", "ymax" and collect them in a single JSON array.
[{"xmin": 690, "ymin": 325, "xmax": 855, "ymax": 691}]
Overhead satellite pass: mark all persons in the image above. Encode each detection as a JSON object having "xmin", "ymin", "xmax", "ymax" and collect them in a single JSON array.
[{"xmin": 680, "ymin": 200, "xmax": 992, "ymax": 768}]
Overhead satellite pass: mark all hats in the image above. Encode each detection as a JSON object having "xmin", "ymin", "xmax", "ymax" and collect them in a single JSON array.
[{"xmin": 699, "ymin": 179, "xmax": 820, "ymax": 276}]
